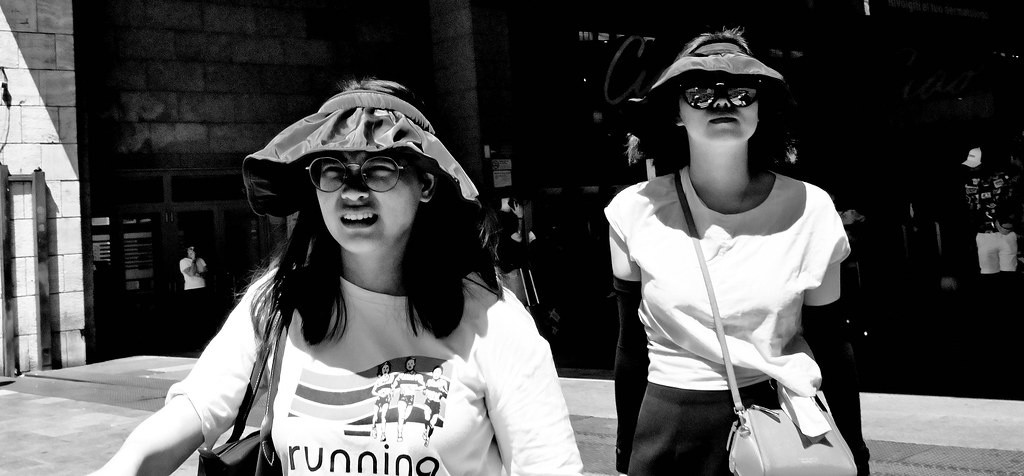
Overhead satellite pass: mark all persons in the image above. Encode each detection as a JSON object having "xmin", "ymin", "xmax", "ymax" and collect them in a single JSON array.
[
  {"xmin": 180, "ymin": 244, "xmax": 208, "ymax": 328},
  {"xmin": 962, "ymin": 147, "xmax": 1017, "ymax": 312},
  {"xmin": 134, "ymin": 78, "xmax": 584, "ymax": 476},
  {"xmin": 604, "ymin": 26, "xmax": 870, "ymax": 476},
  {"xmin": 496, "ymin": 193, "xmax": 526, "ymax": 303}
]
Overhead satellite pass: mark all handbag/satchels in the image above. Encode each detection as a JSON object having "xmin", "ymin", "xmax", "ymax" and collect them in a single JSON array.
[
  {"xmin": 197, "ymin": 430, "xmax": 283, "ymax": 476},
  {"xmin": 727, "ymin": 389, "xmax": 859, "ymax": 476}
]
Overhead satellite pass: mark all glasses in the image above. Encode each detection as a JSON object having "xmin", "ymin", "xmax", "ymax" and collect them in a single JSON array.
[
  {"xmin": 678, "ymin": 76, "xmax": 762, "ymax": 109},
  {"xmin": 304, "ymin": 156, "xmax": 407, "ymax": 194}
]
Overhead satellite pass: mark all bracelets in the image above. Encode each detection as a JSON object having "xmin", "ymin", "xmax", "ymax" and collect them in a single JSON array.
[
  {"xmin": 192, "ymin": 260, "xmax": 195, "ymax": 264},
  {"xmin": 518, "ymin": 217, "xmax": 523, "ymax": 220}
]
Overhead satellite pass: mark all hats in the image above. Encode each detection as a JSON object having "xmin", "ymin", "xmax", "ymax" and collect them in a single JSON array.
[
  {"xmin": 242, "ymin": 88, "xmax": 485, "ymax": 213},
  {"xmin": 647, "ymin": 36, "xmax": 790, "ymax": 94}
]
